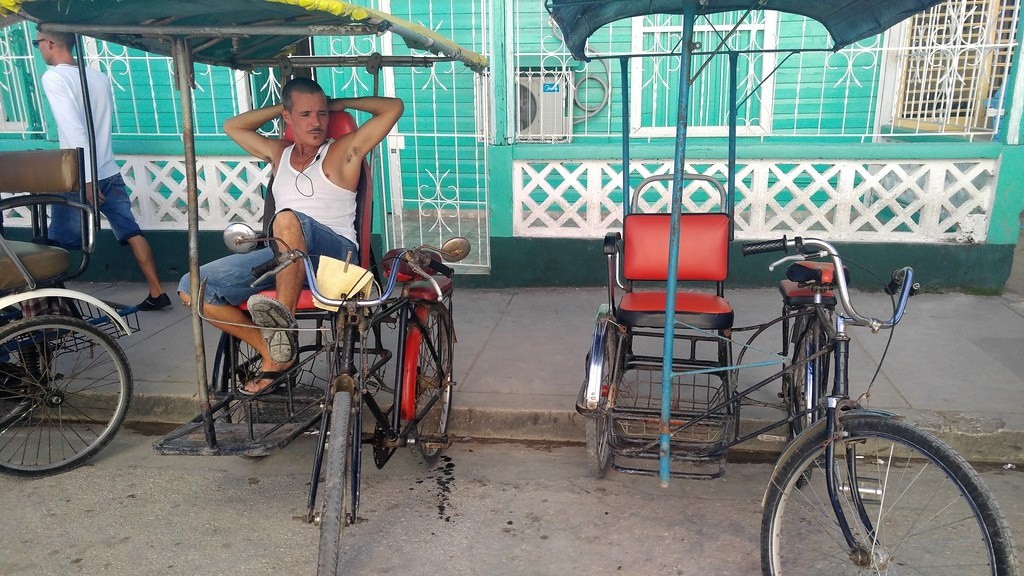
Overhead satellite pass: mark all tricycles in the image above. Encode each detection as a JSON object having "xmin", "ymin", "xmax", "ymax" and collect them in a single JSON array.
[
  {"xmin": 543, "ymin": 3, "xmax": 1013, "ymax": 576},
  {"xmin": 0, "ymin": 22, "xmax": 140, "ymax": 479},
  {"xmin": 0, "ymin": 0, "xmax": 485, "ymax": 575}
]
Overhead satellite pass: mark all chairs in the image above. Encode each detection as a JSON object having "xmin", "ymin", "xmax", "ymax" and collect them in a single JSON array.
[
  {"xmin": 0, "ymin": 147, "xmax": 89, "ymax": 295},
  {"xmin": 602, "ymin": 172, "xmax": 735, "ymax": 415},
  {"xmin": 212, "ymin": 109, "xmax": 373, "ymax": 394}
]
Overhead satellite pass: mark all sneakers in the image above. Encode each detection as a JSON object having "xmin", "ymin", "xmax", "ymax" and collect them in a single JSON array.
[{"xmin": 136, "ymin": 291, "xmax": 171, "ymax": 311}]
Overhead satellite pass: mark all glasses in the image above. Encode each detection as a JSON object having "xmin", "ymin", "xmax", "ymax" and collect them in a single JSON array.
[{"xmin": 32, "ymin": 39, "xmax": 54, "ymax": 48}]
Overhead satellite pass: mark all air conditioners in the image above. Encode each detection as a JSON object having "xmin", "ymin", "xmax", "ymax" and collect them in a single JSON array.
[{"xmin": 478, "ymin": 76, "xmax": 565, "ymax": 139}]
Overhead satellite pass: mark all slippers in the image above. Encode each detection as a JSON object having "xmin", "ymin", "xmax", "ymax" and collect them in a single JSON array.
[
  {"xmin": 247, "ymin": 294, "xmax": 299, "ymax": 363},
  {"xmin": 238, "ymin": 365, "xmax": 297, "ymax": 396}
]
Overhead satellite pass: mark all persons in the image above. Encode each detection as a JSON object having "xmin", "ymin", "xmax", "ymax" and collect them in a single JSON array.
[
  {"xmin": 36, "ymin": 23, "xmax": 174, "ymax": 312},
  {"xmin": 175, "ymin": 76, "xmax": 404, "ymax": 396}
]
